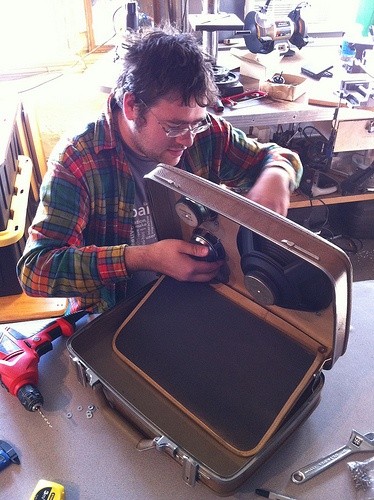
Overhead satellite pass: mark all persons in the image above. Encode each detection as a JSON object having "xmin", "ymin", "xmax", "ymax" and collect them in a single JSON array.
[{"xmin": 16, "ymin": 30, "xmax": 303, "ymax": 317}]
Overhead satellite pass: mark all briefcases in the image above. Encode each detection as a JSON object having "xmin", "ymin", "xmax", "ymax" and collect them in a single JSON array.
[{"xmin": 67, "ymin": 164, "xmax": 354, "ymax": 500}]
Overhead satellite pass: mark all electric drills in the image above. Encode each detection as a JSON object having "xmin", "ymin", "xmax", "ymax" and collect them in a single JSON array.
[{"xmin": 0, "ymin": 306, "xmax": 87, "ymax": 430}]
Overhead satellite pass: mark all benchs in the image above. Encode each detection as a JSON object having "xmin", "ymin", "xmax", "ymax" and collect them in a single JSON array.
[{"xmin": 206, "ymin": 45, "xmax": 374, "ymax": 209}]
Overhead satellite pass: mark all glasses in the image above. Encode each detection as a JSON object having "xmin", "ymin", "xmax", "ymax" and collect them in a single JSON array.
[{"xmin": 138, "ymin": 97, "xmax": 212, "ymax": 137}]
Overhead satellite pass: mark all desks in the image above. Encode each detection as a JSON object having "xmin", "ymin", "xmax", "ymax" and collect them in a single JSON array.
[{"xmin": 0, "ymin": 276, "xmax": 374, "ymax": 500}]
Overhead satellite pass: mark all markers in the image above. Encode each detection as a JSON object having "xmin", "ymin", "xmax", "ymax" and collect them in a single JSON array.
[{"xmin": 255, "ymin": 488, "xmax": 297, "ymax": 500}]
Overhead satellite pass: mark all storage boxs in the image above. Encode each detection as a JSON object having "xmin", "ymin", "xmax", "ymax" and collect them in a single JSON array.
[{"xmin": 264, "ymin": 73, "xmax": 307, "ymax": 102}]
